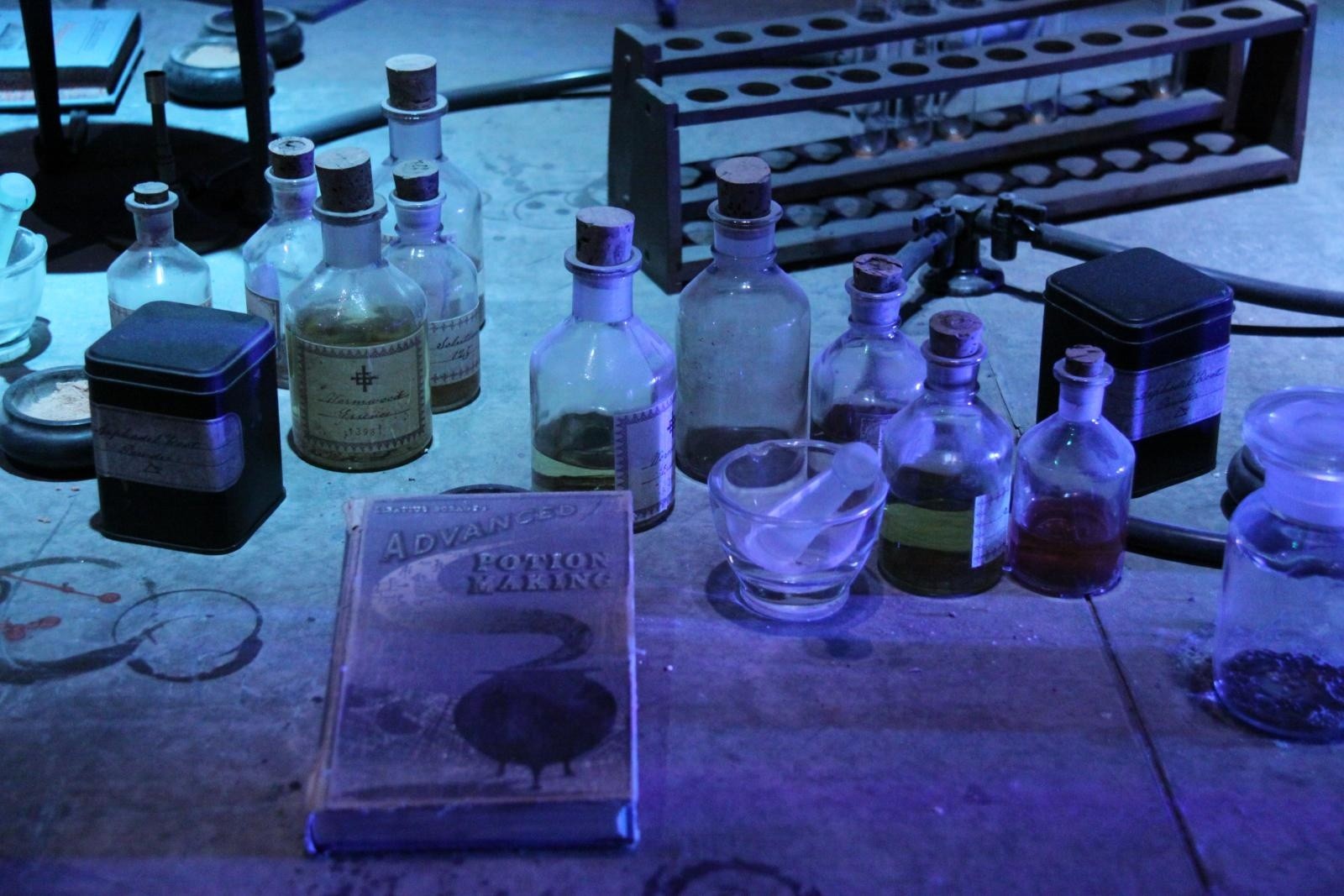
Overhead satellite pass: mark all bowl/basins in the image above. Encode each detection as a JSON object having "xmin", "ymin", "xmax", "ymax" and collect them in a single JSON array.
[
  {"xmin": 704, "ymin": 438, "xmax": 887, "ymax": 620},
  {"xmin": 0, "ymin": 226, "xmax": 50, "ymax": 364}
]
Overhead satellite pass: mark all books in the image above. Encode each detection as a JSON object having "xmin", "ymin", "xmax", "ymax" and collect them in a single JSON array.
[{"xmin": 301, "ymin": 492, "xmax": 641, "ymax": 857}]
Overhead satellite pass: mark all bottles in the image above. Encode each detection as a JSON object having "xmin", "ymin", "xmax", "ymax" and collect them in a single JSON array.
[{"xmin": 104, "ymin": 51, "xmax": 1341, "ymax": 744}]
